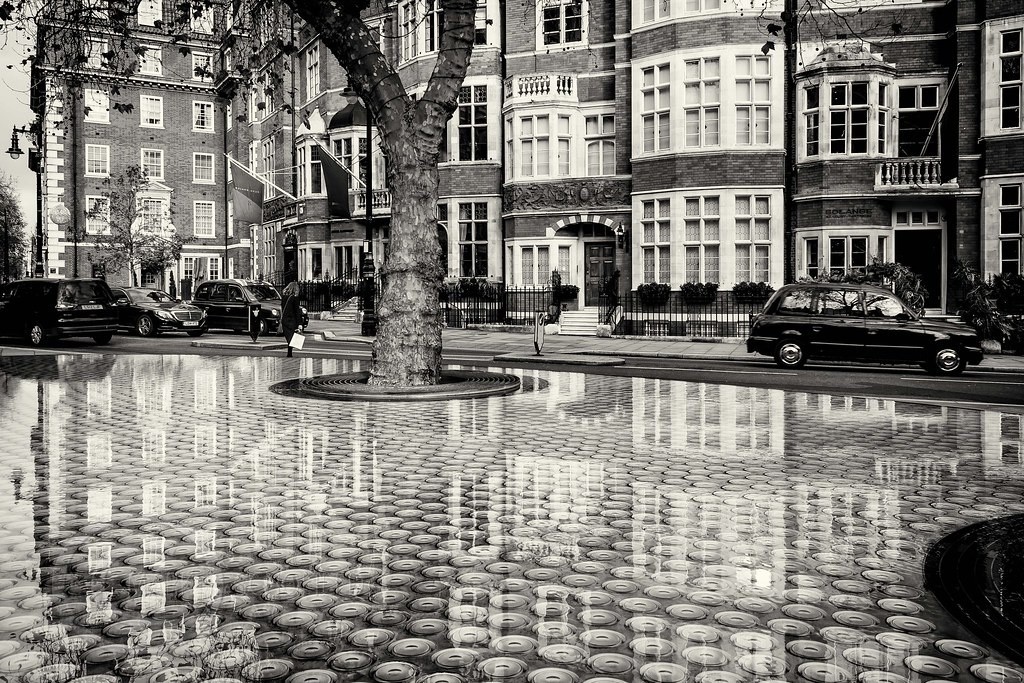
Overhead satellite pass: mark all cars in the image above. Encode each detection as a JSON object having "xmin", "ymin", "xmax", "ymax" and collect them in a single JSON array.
[{"xmin": 109, "ymin": 287, "xmax": 208, "ymax": 337}]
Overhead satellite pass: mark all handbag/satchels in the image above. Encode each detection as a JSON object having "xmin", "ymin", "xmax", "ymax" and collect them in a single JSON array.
[
  {"xmin": 277, "ymin": 320, "xmax": 283, "ymax": 334},
  {"xmin": 288, "ymin": 329, "xmax": 305, "ymax": 350}
]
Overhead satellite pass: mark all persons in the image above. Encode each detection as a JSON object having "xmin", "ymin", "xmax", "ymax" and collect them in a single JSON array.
[{"xmin": 281, "ymin": 282, "xmax": 302, "ymax": 358}]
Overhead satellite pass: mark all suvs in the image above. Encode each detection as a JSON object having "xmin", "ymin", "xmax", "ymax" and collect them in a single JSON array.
[
  {"xmin": 747, "ymin": 283, "xmax": 984, "ymax": 377},
  {"xmin": 190, "ymin": 279, "xmax": 309, "ymax": 334},
  {"xmin": 0, "ymin": 278, "xmax": 119, "ymax": 348}
]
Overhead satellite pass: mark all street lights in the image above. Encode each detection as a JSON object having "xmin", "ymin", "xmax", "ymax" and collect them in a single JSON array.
[
  {"xmin": 340, "ymin": 75, "xmax": 377, "ymax": 337},
  {"xmin": 5, "ymin": 125, "xmax": 44, "ymax": 278}
]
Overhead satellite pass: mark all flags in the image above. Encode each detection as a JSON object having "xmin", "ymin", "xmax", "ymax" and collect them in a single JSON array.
[
  {"xmin": 230, "ymin": 160, "xmax": 263, "ymax": 224},
  {"xmin": 319, "ymin": 147, "xmax": 351, "ymax": 220}
]
